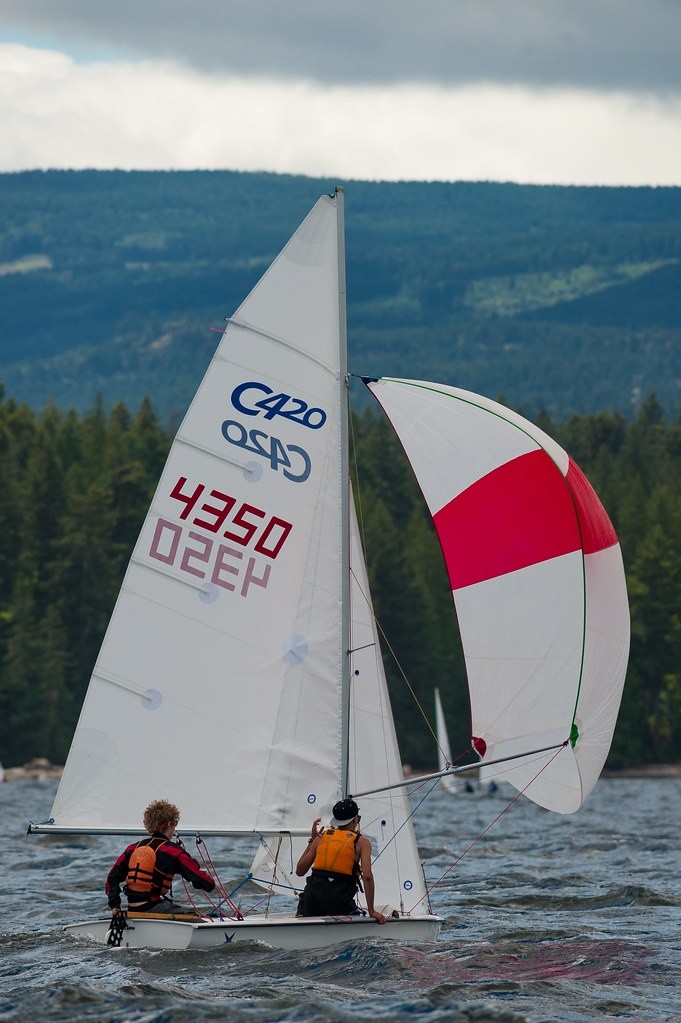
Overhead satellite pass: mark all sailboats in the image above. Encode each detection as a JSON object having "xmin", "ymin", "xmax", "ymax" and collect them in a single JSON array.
[
  {"xmin": 432, "ymin": 686, "xmax": 498, "ymax": 802},
  {"xmin": 24, "ymin": 173, "xmax": 631, "ymax": 958}
]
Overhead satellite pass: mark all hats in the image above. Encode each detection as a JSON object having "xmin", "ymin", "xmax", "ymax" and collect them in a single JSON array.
[{"xmin": 330, "ymin": 800, "xmax": 359, "ymax": 826}]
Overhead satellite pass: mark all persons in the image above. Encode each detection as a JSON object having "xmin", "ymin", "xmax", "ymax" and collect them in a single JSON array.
[
  {"xmin": 295, "ymin": 799, "xmax": 386, "ymax": 925},
  {"xmin": 487, "ymin": 780, "xmax": 497, "ymax": 794},
  {"xmin": 104, "ymin": 799, "xmax": 216, "ymax": 916},
  {"xmin": 464, "ymin": 780, "xmax": 473, "ymax": 793}
]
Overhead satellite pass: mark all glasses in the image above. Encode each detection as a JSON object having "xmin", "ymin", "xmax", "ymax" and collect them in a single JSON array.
[{"xmin": 167, "ymin": 818, "xmax": 179, "ymax": 827}]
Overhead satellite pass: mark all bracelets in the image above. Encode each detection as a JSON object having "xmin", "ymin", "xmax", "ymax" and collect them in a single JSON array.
[{"xmin": 309, "ymin": 839, "xmax": 313, "ymax": 843}]
